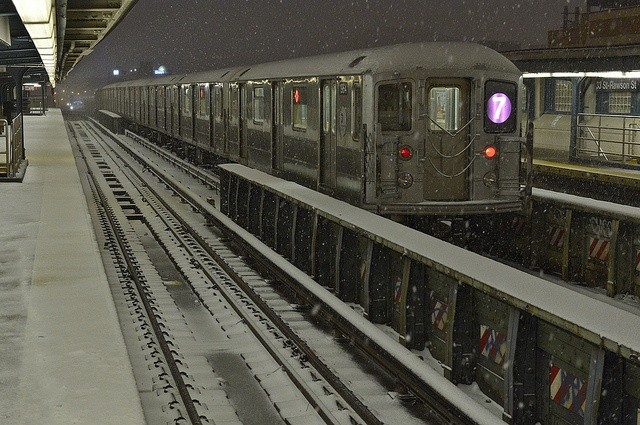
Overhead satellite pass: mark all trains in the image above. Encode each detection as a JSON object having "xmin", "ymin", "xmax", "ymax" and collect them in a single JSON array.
[{"xmin": 70, "ymin": 41, "xmax": 536, "ymax": 218}]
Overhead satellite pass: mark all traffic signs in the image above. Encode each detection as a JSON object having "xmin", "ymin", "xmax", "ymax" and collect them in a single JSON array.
[{"xmin": 594, "ymin": 78, "xmax": 640, "ymax": 92}]
